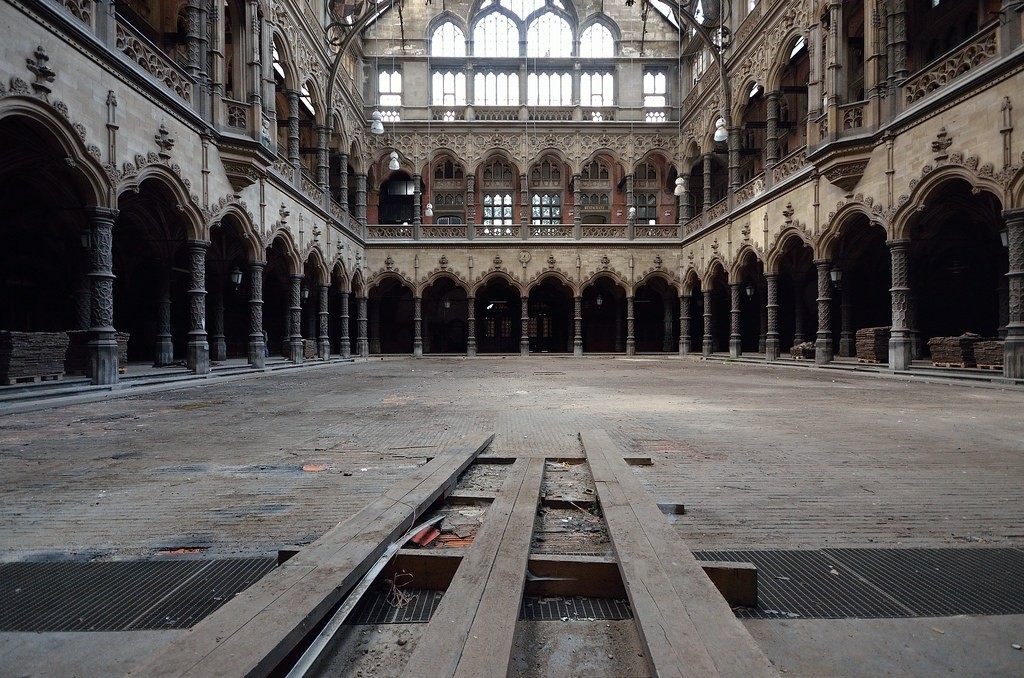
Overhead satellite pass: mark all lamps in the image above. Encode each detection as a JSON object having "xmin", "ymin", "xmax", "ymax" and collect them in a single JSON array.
[
  {"xmin": 714, "ymin": 0, "xmax": 729, "ymax": 142},
  {"xmin": 596, "ymin": 294, "xmax": 603, "ymax": 310},
  {"xmin": 746, "ymin": 283, "xmax": 755, "ymax": 302},
  {"xmin": 830, "ymin": 264, "xmax": 847, "ymax": 288},
  {"xmin": 388, "ymin": 0, "xmax": 400, "ymax": 171},
  {"xmin": 998, "ymin": 228, "xmax": 1009, "ymax": 247},
  {"xmin": 629, "ymin": 5, "xmax": 637, "ymax": 218},
  {"xmin": 444, "ymin": 298, "xmax": 451, "ymax": 309},
  {"xmin": 425, "ymin": 0, "xmax": 434, "ymax": 217},
  {"xmin": 371, "ymin": 0, "xmax": 385, "ymax": 135},
  {"xmin": 674, "ymin": 0, "xmax": 686, "ymax": 196},
  {"xmin": 301, "ymin": 285, "xmax": 310, "ymax": 303},
  {"xmin": 231, "ymin": 266, "xmax": 244, "ymax": 295}
]
